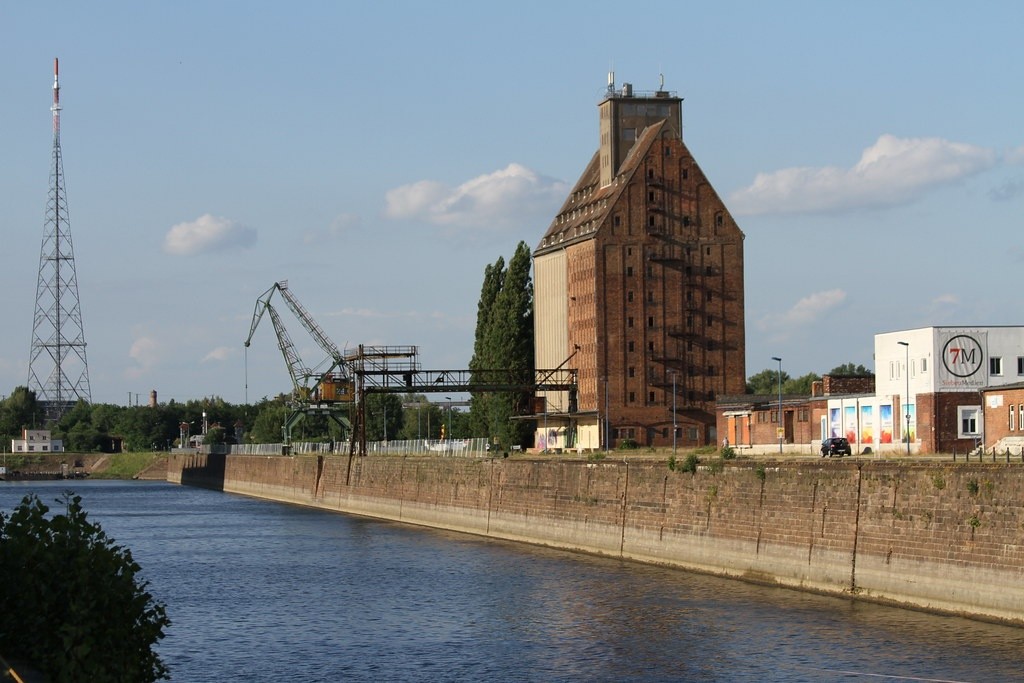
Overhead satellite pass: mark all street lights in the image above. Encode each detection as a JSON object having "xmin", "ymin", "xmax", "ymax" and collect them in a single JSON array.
[
  {"xmin": 417, "ymin": 401, "xmax": 421, "ymax": 455},
  {"xmin": 600, "ymin": 378, "xmax": 609, "ymax": 452},
  {"xmin": 898, "ymin": 342, "xmax": 910, "ymax": 456},
  {"xmin": 445, "ymin": 396, "xmax": 451, "ymax": 458},
  {"xmin": 539, "ymin": 385, "xmax": 547, "ymax": 452},
  {"xmin": 667, "ymin": 369, "xmax": 677, "ymax": 454},
  {"xmin": 771, "ymin": 356, "xmax": 782, "ymax": 453},
  {"xmin": 372, "ymin": 412, "xmax": 387, "ymax": 456}
]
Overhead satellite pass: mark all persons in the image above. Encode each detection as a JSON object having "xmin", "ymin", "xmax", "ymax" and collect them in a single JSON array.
[{"xmin": 722, "ymin": 437, "xmax": 729, "ymax": 448}]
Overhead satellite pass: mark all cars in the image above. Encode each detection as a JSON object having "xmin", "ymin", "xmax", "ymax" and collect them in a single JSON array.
[
  {"xmin": 820, "ymin": 437, "xmax": 851, "ymax": 457},
  {"xmin": 485, "ymin": 443, "xmax": 490, "ymax": 451}
]
